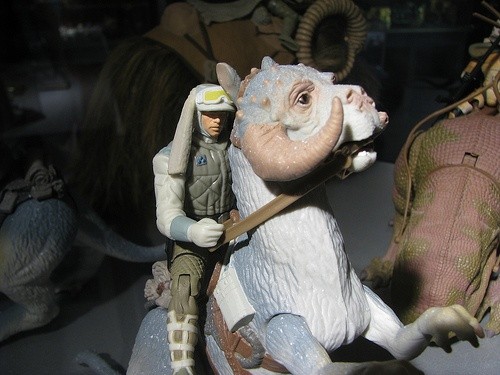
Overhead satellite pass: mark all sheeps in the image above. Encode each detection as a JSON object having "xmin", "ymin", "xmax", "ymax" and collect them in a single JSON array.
[{"xmin": 76, "ymin": 55, "xmax": 485, "ymax": 375}]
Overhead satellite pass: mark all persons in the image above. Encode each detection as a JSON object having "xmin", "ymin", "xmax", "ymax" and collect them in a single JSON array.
[{"xmin": 151, "ymin": 84, "xmax": 239, "ymax": 374}]
